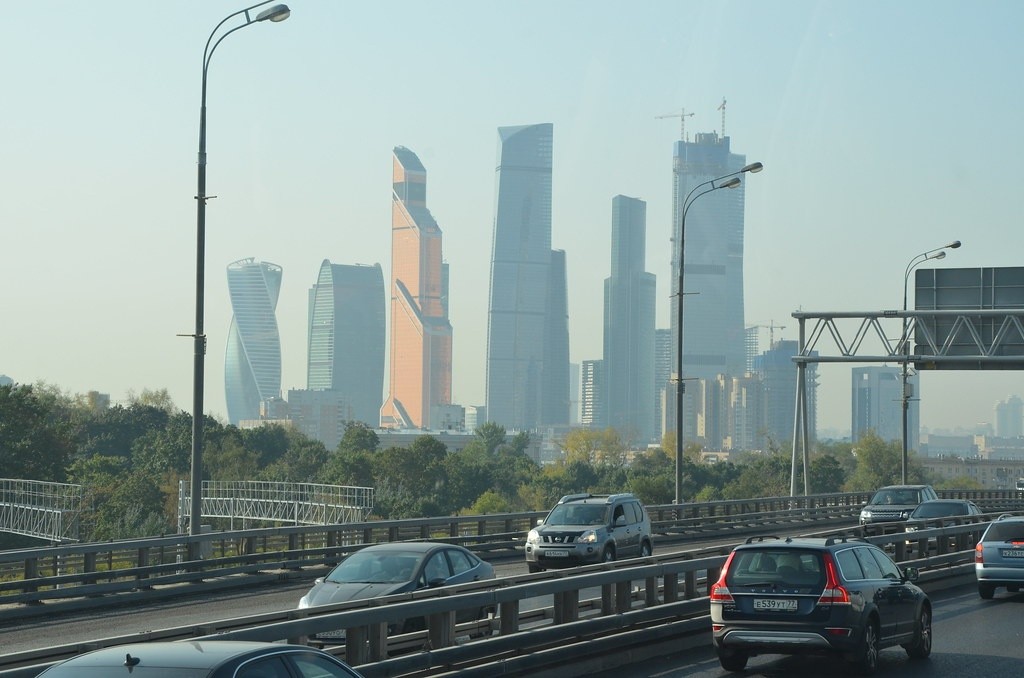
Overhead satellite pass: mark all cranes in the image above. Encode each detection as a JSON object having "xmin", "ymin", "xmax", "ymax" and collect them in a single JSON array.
[
  {"xmin": 716, "ymin": 95, "xmax": 727, "ymax": 138},
  {"xmin": 654, "ymin": 107, "xmax": 696, "ymax": 142}
]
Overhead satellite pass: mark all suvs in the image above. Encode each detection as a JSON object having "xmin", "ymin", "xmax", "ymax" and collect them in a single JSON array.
[
  {"xmin": 858, "ymin": 484, "xmax": 939, "ymax": 537},
  {"xmin": 525, "ymin": 493, "xmax": 655, "ymax": 575},
  {"xmin": 903, "ymin": 498, "xmax": 984, "ymax": 548},
  {"xmin": 708, "ymin": 533, "xmax": 933, "ymax": 675}
]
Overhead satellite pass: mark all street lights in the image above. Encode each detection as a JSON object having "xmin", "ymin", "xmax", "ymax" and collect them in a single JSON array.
[
  {"xmin": 182, "ymin": 1, "xmax": 293, "ymax": 581},
  {"xmin": 671, "ymin": 159, "xmax": 766, "ymax": 517},
  {"xmin": 899, "ymin": 238, "xmax": 964, "ymax": 484}
]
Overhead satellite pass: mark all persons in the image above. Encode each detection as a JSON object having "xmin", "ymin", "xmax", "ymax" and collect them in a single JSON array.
[{"xmin": 760, "ymin": 556, "xmax": 777, "ymax": 573}]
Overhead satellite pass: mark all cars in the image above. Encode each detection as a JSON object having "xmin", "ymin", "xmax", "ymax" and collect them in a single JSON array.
[
  {"xmin": 974, "ymin": 514, "xmax": 1024, "ymax": 598},
  {"xmin": 32, "ymin": 641, "xmax": 370, "ymax": 678},
  {"xmin": 295, "ymin": 539, "xmax": 499, "ymax": 661}
]
